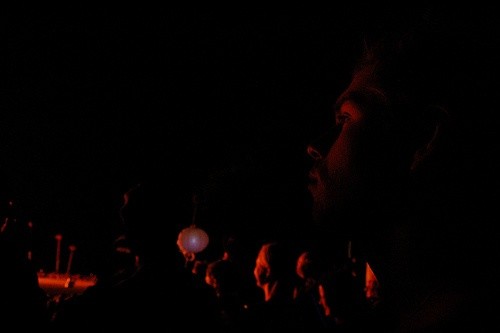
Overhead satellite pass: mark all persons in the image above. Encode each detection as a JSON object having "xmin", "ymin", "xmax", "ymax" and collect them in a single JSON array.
[
  {"xmin": 0, "ymin": 199, "xmax": 384, "ymax": 333},
  {"xmin": 309, "ymin": 26, "xmax": 500, "ymax": 332}
]
